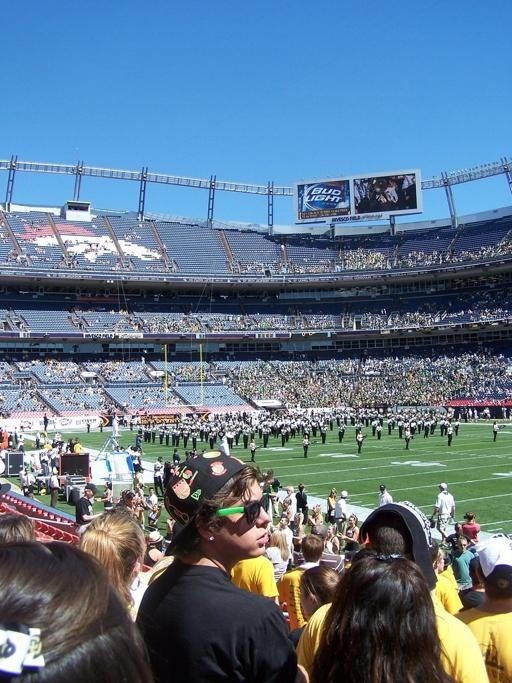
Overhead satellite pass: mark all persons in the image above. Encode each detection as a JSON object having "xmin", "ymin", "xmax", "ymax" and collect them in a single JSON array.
[
  {"xmin": 1, "ymin": 212, "xmax": 144, "ymax": 270},
  {"xmin": 1, "ymin": 283, "xmax": 510, "ymax": 461},
  {"xmin": 355, "ymin": 173, "xmax": 417, "ymax": 212},
  {"xmin": 231, "ymin": 228, "xmax": 510, "ymax": 272},
  {"xmin": 0, "ymin": 447, "xmax": 511, "ymax": 683}
]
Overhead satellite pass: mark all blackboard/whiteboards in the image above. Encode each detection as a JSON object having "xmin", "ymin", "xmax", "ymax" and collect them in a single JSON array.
[{"xmin": 59, "ymin": 453, "xmax": 90, "ymax": 477}]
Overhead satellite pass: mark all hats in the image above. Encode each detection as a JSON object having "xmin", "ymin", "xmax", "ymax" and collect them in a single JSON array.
[
  {"xmin": 341, "ymin": 490, "xmax": 347, "ymax": 497},
  {"xmin": 148, "ymin": 530, "xmax": 164, "ymax": 544},
  {"xmin": 474, "ymin": 536, "xmax": 512, "ymax": 578},
  {"xmin": 163, "ymin": 449, "xmax": 249, "ymax": 557},
  {"xmin": 85, "ymin": 483, "xmax": 97, "ymax": 491},
  {"xmin": 380, "ymin": 484, "xmax": 386, "ymax": 490},
  {"xmin": 439, "ymin": 483, "xmax": 447, "ymax": 489}
]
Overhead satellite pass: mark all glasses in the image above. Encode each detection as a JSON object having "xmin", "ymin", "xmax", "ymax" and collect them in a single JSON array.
[{"xmin": 213, "ymin": 492, "xmax": 271, "ymax": 529}]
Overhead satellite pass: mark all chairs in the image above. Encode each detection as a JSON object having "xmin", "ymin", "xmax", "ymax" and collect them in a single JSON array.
[{"xmin": 0, "ymin": 482, "xmax": 158, "ymax": 575}]
